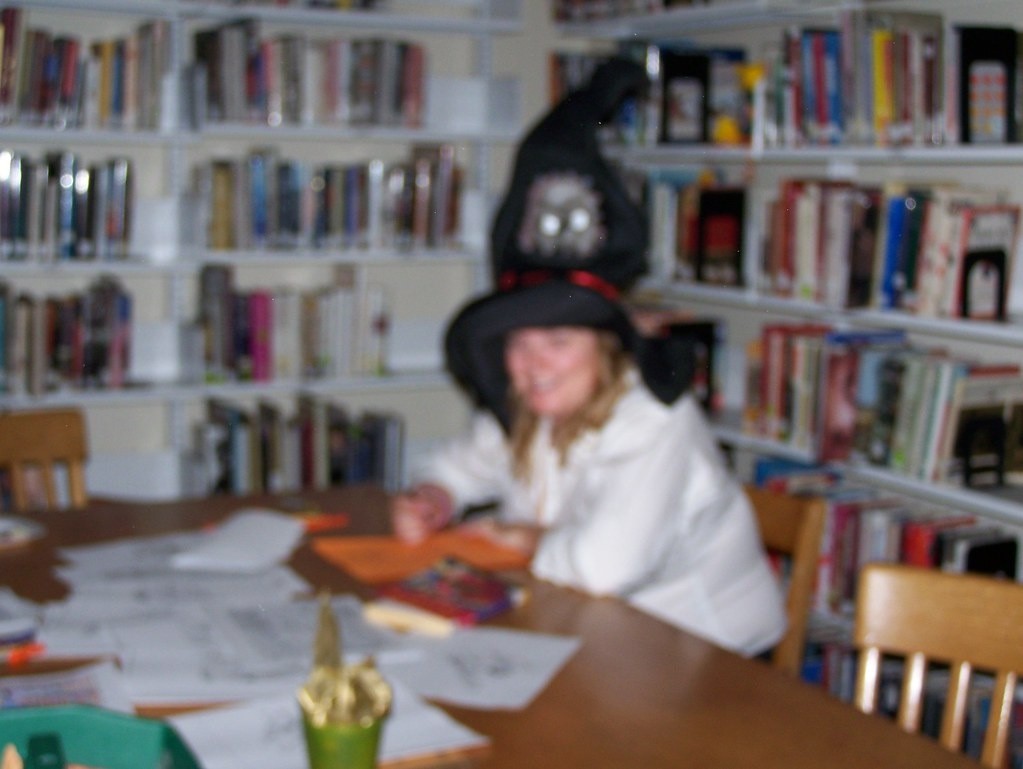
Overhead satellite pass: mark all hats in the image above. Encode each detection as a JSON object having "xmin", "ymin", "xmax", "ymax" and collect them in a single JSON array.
[{"xmin": 438, "ymin": 47, "xmax": 698, "ymax": 424}]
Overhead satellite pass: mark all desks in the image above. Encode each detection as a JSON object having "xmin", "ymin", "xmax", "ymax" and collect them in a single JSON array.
[{"xmin": 2, "ymin": 495, "xmax": 989, "ymax": 769}]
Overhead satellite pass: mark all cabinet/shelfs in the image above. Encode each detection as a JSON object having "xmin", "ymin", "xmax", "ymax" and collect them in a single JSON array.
[
  {"xmin": 546, "ymin": 0, "xmax": 1021, "ymax": 768},
  {"xmin": 2, "ymin": 0, "xmax": 528, "ymax": 501}
]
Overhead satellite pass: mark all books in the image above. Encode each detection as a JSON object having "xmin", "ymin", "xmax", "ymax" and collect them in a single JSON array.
[{"xmin": 0, "ymin": 0, "xmax": 1023, "ymax": 769}]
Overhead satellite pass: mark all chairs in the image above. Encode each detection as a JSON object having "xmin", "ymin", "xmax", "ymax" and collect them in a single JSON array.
[
  {"xmin": 742, "ymin": 486, "xmax": 826, "ymax": 676},
  {"xmin": 853, "ymin": 565, "xmax": 1023, "ymax": 769},
  {"xmin": 0, "ymin": 408, "xmax": 87, "ymax": 509}
]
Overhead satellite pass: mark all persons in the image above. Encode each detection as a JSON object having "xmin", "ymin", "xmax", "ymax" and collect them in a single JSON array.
[{"xmin": 390, "ymin": 266, "xmax": 790, "ymax": 666}]
